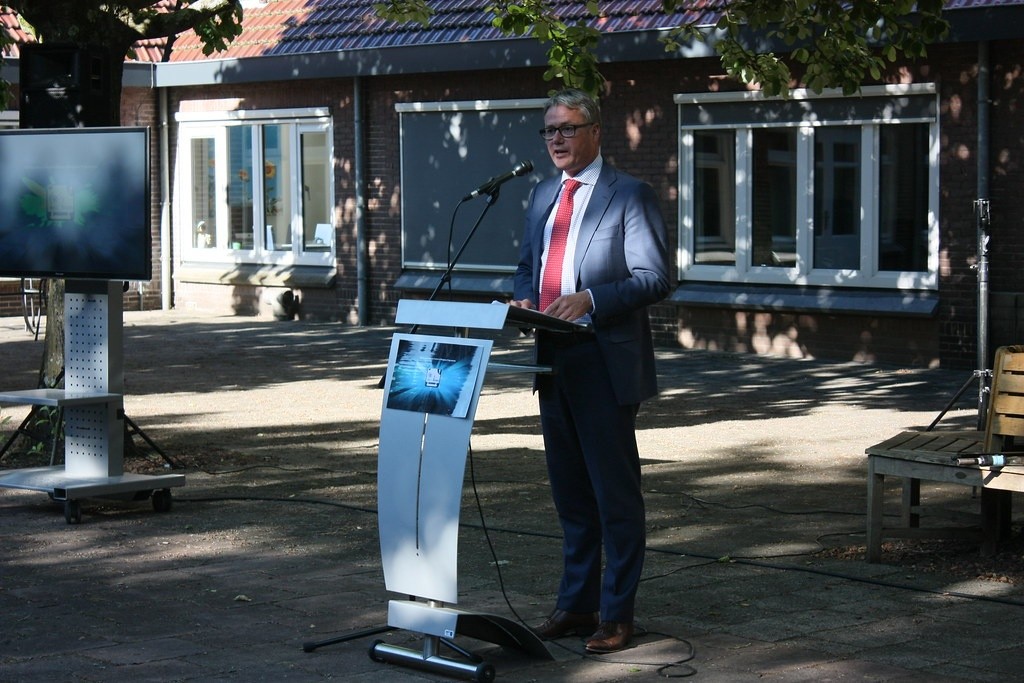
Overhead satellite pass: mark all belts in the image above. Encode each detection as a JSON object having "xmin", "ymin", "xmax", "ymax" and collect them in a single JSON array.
[{"xmin": 538, "ymin": 329, "xmax": 597, "ymax": 345}]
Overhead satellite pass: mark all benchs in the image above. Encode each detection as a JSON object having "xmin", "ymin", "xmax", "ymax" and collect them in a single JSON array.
[{"xmin": 865, "ymin": 344, "xmax": 1024, "ymax": 564}]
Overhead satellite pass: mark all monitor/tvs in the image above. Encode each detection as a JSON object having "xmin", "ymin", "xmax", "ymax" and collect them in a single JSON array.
[{"xmin": 0, "ymin": 126, "xmax": 152, "ymax": 282}]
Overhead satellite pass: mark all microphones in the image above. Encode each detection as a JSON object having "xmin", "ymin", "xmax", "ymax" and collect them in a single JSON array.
[{"xmin": 462, "ymin": 159, "xmax": 535, "ymax": 203}]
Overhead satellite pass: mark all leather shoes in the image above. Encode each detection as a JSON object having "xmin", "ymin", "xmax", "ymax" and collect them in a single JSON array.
[
  {"xmin": 531, "ymin": 608, "xmax": 600, "ymax": 641},
  {"xmin": 585, "ymin": 620, "xmax": 633, "ymax": 654}
]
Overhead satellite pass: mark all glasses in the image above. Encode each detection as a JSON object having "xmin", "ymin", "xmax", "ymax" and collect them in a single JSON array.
[{"xmin": 540, "ymin": 122, "xmax": 592, "ymax": 140}]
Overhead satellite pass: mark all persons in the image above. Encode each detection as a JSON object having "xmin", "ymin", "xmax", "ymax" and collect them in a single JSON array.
[{"xmin": 502, "ymin": 92, "xmax": 680, "ymax": 653}]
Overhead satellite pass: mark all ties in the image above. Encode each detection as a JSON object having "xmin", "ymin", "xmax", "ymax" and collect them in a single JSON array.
[{"xmin": 539, "ymin": 180, "xmax": 582, "ymax": 313}]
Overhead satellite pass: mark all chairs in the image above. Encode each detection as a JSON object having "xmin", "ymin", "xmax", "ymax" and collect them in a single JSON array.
[{"xmin": 232, "ymin": 221, "xmax": 333, "ymax": 252}]
